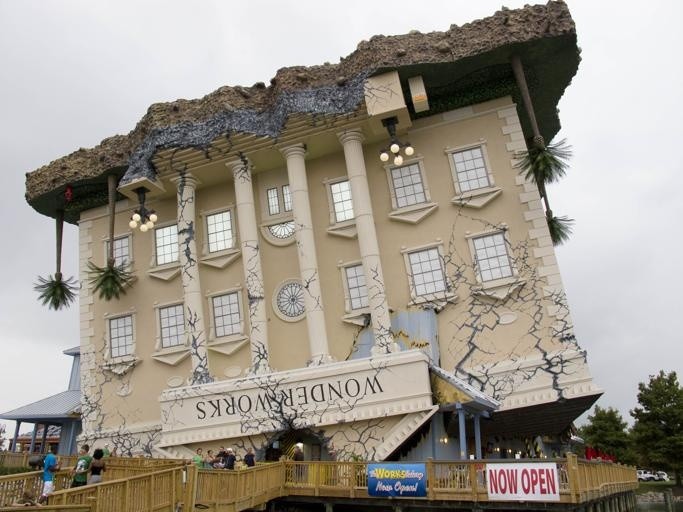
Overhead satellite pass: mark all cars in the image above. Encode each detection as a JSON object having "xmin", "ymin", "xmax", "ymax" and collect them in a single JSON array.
[{"xmin": 637, "ymin": 470, "xmax": 671, "ymax": 481}]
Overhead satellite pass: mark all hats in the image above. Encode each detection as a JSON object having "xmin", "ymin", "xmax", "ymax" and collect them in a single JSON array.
[
  {"xmin": 223, "ymin": 448, "xmax": 235, "ymax": 456},
  {"xmin": 292, "ymin": 443, "xmax": 303, "ymax": 453}
]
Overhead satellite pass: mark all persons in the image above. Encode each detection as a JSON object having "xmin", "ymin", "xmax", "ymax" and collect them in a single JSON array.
[
  {"xmin": 70, "ymin": 444, "xmax": 92, "ymax": 488},
  {"xmin": 10, "ymin": 490, "xmax": 42, "ymax": 506},
  {"xmin": 38, "ymin": 445, "xmax": 62, "ymax": 505},
  {"xmin": 87, "ymin": 448, "xmax": 107, "ymax": 484},
  {"xmin": 192, "ymin": 446, "xmax": 256, "ymax": 470},
  {"xmin": 292, "ymin": 447, "xmax": 303, "ymax": 461}
]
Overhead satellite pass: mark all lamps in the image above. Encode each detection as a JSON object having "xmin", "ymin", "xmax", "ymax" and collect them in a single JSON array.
[
  {"xmin": 380, "ymin": 117, "xmax": 414, "ymax": 166},
  {"xmin": 129, "ymin": 186, "xmax": 158, "ymax": 232}
]
